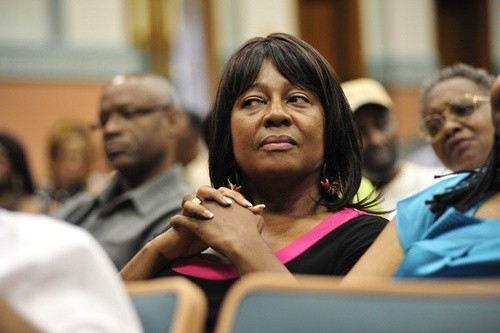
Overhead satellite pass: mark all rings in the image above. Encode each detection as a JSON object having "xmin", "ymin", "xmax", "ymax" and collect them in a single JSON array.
[{"xmin": 193, "ymin": 198, "xmax": 201, "ymax": 204}]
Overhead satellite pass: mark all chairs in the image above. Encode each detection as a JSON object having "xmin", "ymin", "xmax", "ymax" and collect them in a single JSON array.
[
  {"xmin": 214, "ymin": 273, "xmax": 500, "ymax": 333},
  {"xmin": 124, "ymin": 278, "xmax": 208, "ymax": 333}
]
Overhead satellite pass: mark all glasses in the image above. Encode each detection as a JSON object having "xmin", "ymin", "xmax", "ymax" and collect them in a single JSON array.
[
  {"xmin": 417, "ymin": 94, "xmax": 490, "ymax": 143},
  {"xmin": 89, "ymin": 104, "xmax": 170, "ymax": 132}
]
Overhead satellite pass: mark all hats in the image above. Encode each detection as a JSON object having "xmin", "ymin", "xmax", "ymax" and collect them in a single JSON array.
[{"xmin": 340, "ymin": 78, "xmax": 392, "ymax": 114}]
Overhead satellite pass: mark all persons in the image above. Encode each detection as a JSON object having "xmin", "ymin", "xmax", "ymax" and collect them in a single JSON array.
[
  {"xmin": 118, "ymin": 33, "xmax": 390, "ymax": 333},
  {"xmin": 339, "ymin": 64, "xmax": 495, "ymax": 221},
  {"xmin": 0, "ymin": 72, "xmax": 212, "ymax": 271},
  {"xmin": 342, "ymin": 72, "xmax": 500, "ymax": 278},
  {"xmin": 0, "ymin": 206, "xmax": 146, "ymax": 333}
]
